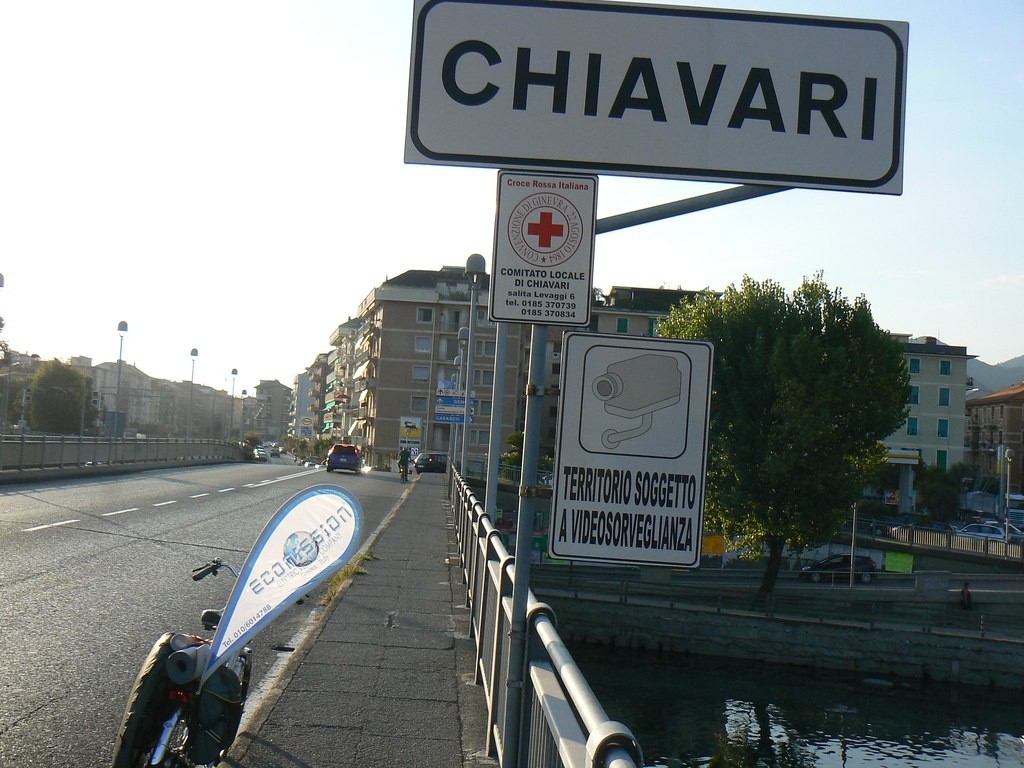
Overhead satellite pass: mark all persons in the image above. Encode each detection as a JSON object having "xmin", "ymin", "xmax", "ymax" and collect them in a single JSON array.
[{"xmin": 397, "ymin": 447, "xmax": 411, "ymax": 482}]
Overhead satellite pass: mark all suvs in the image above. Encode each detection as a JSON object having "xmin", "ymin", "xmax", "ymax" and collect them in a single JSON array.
[{"xmin": 800, "ymin": 553, "xmax": 877, "ymax": 584}]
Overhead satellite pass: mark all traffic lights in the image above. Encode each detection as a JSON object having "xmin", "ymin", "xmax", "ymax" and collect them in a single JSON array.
[
  {"xmin": 24, "ymin": 387, "xmax": 31, "ymax": 405},
  {"xmin": 91, "ymin": 391, "xmax": 99, "ymax": 407}
]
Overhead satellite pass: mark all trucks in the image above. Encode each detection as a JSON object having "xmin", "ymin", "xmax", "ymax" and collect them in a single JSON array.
[
  {"xmin": 83, "ymin": 410, "xmax": 126, "ymax": 438},
  {"xmin": 995, "ymin": 493, "xmax": 1024, "ymax": 528}
]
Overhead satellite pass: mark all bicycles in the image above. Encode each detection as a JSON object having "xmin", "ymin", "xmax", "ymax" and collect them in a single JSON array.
[
  {"xmin": 397, "ymin": 460, "xmax": 414, "ymax": 484},
  {"xmin": 137, "ymin": 557, "xmax": 308, "ymax": 767}
]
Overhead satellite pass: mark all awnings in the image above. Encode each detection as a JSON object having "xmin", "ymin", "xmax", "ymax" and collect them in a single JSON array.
[{"xmin": 348, "ymin": 422, "xmax": 362, "ymax": 436}]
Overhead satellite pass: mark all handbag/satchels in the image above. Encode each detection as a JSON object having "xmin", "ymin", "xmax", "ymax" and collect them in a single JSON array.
[{"xmin": 188, "ymin": 664, "xmax": 243, "ymax": 764}]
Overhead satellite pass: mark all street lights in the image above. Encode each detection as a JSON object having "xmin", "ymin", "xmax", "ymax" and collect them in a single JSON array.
[
  {"xmin": 3, "ymin": 352, "xmax": 41, "ymax": 434},
  {"xmin": 1003, "ymin": 445, "xmax": 1016, "ymax": 545},
  {"xmin": 449, "ymin": 325, "xmax": 472, "ymax": 480},
  {"xmin": 228, "ymin": 368, "xmax": 237, "ymax": 441},
  {"xmin": 113, "ymin": 321, "xmax": 129, "ymax": 437},
  {"xmin": 461, "ymin": 251, "xmax": 488, "ymax": 481},
  {"xmin": 186, "ymin": 346, "xmax": 200, "ymax": 439},
  {"xmin": 240, "ymin": 390, "xmax": 247, "ymax": 443}
]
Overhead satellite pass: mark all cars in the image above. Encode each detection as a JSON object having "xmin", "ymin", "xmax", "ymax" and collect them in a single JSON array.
[
  {"xmin": 414, "ymin": 452, "xmax": 461, "ymax": 474},
  {"xmin": 866, "ymin": 512, "xmax": 929, "ymax": 536},
  {"xmin": 957, "ymin": 524, "xmax": 1006, "ymax": 545},
  {"xmin": 324, "ymin": 444, "xmax": 363, "ymax": 476},
  {"xmin": 263, "ymin": 440, "xmax": 288, "ymax": 454},
  {"xmin": 890, "ymin": 521, "xmax": 957, "ymax": 535},
  {"xmin": 270, "ymin": 449, "xmax": 281, "ymax": 458},
  {"xmin": 986, "ymin": 520, "xmax": 1024, "ymax": 545},
  {"xmin": 253, "ymin": 444, "xmax": 267, "ymax": 461}
]
